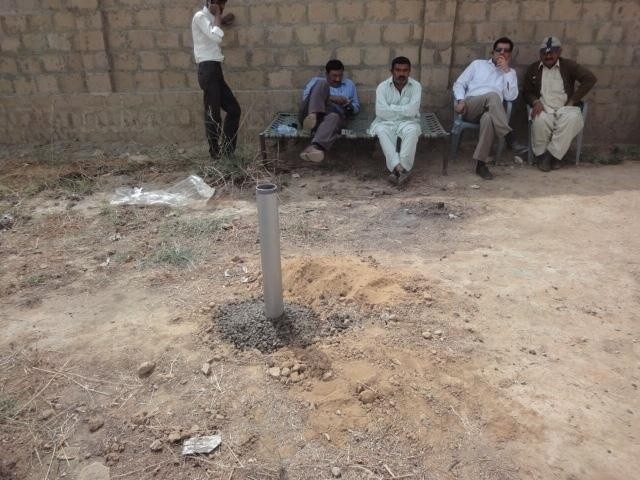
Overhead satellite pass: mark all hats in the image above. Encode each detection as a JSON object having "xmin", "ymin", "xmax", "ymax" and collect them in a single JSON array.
[{"xmin": 539, "ymin": 36, "xmax": 562, "ymax": 55}]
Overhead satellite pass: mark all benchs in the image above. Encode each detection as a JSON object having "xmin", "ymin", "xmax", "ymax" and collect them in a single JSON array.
[{"xmin": 259, "ymin": 111, "xmax": 452, "ymax": 175}]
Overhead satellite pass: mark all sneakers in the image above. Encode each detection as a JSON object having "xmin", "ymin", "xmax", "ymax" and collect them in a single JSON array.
[
  {"xmin": 387, "ymin": 169, "xmax": 413, "ymax": 186},
  {"xmin": 536, "ymin": 151, "xmax": 562, "ymax": 172},
  {"xmin": 475, "ymin": 167, "xmax": 493, "ymax": 180},
  {"xmin": 299, "ymin": 145, "xmax": 325, "ymax": 163},
  {"xmin": 508, "ymin": 139, "xmax": 530, "ymax": 155},
  {"xmin": 301, "ymin": 113, "xmax": 317, "ymax": 138}
]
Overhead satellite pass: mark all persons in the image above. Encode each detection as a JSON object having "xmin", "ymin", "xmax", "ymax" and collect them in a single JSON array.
[
  {"xmin": 522, "ymin": 35, "xmax": 599, "ymax": 173},
  {"xmin": 295, "ymin": 59, "xmax": 361, "ymax": 163},
  {"xmin": 366, "ymin": 56, "xmax": 423, "ymax": 187},
  {"xmin": 451, "ymin": 37, "xmax": 530, "ymax": 181},
  {"xmin": 190, "ymin": 0, "xmax": 241, "ymax": 162}
]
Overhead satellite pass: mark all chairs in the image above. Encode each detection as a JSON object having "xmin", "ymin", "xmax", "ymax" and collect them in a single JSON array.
[{"xmin": 448, "ymin": 88, "xmax": 588, "ymax": 169}]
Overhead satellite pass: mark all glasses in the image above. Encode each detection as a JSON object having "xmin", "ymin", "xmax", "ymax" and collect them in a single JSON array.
[{"xmin": 495, "ymin": 48, "xmax": 511, "ymax": 52}]
